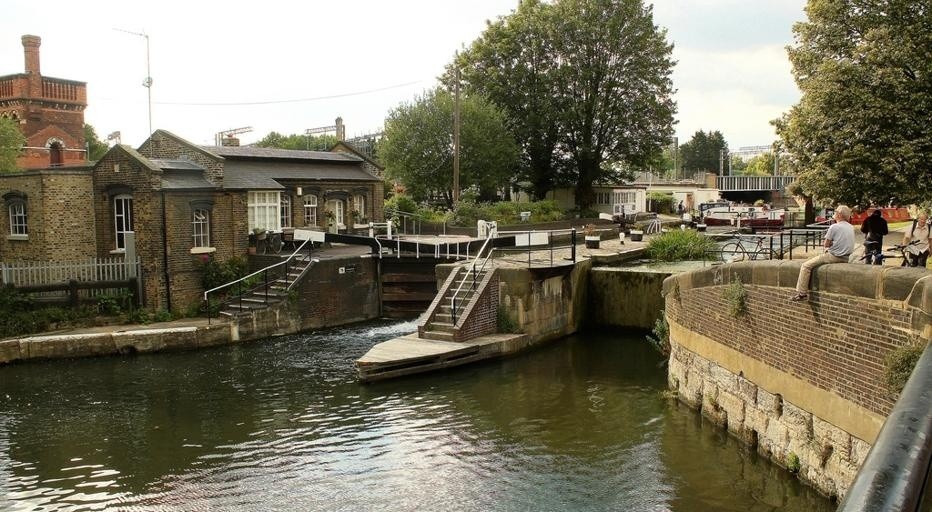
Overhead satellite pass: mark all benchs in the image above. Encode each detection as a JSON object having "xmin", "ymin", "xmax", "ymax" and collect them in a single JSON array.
[{"xmin": 280, "ymin": 226, "xmax": 324, "ymax": 252}]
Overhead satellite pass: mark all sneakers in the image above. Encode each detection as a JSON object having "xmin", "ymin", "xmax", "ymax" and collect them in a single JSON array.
[{"xmin": 789, "ymin": 290, "xmax": 808, "ymax": 302}]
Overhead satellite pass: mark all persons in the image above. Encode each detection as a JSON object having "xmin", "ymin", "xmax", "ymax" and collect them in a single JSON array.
[
  {"xmin": 679, "ymin": 200, "xmax": 685, "ymax": 218},
  {"xmin": 905, "ymin": 213, "xmax": 932, "ymax": 268},
  {"xmin": 861, "ymin": 210, "xmax": 888, "ymax": 265},
  {"xmin": 791, "ymin": 205, "xmax": 855, "ymax": 301}
]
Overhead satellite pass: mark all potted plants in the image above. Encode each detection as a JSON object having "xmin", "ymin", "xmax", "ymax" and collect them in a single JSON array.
[
  {"xmin": 630, "ymin": 223, "xmax": 644, "ymax": 241},
  {"xmin": 697, "ymin": 216, "xmax": 708, "ymax": 232},
  {"xmin": 583, "ymin": 223, "xmax": 602, "ymax": 248},
  {"xmin": 661, "ymin": 221, "xmax": 674, "ymax": 236}
]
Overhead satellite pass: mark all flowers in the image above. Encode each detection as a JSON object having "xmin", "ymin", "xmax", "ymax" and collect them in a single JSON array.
[
  {"xmin": 320, "ymin": 206, "xmax": 336, "ymax": 222},
  {"xmin": 350, "ymin": 209, "xmax": 360, "ymax": 217}
]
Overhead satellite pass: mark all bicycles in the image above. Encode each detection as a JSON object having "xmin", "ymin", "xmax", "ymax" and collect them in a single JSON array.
[
  {"xmin": 255, "ymin": 230, "xmax": 282, "ymax": 255},
  {"xmin": 721, "ymin": 232, "xmax": 779, "ymax": 264},
  {"xmin": 863, "ymin": 239, "xmax": 920, "ymax": 267}
]
